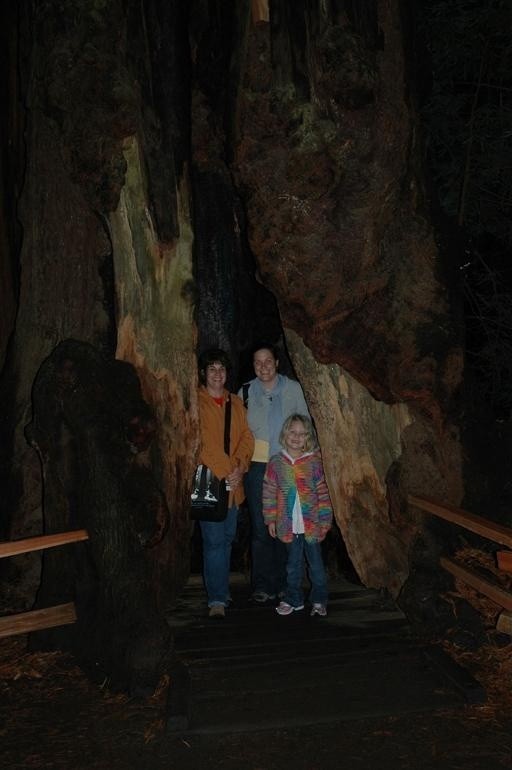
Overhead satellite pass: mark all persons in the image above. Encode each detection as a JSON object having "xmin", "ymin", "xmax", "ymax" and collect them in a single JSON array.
[{"xmin": 197, "ymin": 345, "xmax": 333, "ymax": 617}]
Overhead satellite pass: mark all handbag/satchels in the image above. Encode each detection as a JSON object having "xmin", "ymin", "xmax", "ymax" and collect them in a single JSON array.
[{"xmin": 189, "ymin": 464, "xmax": 228, "ymax": 522}]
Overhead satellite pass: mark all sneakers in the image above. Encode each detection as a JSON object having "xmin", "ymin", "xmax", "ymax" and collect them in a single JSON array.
[
  {"xmin": 251, "ymin": 591, "xmax": 327, "ymax": 616},
  {"xmin": 208, "ymin": 604, "xmax": 225, "ymax": 618}
]
